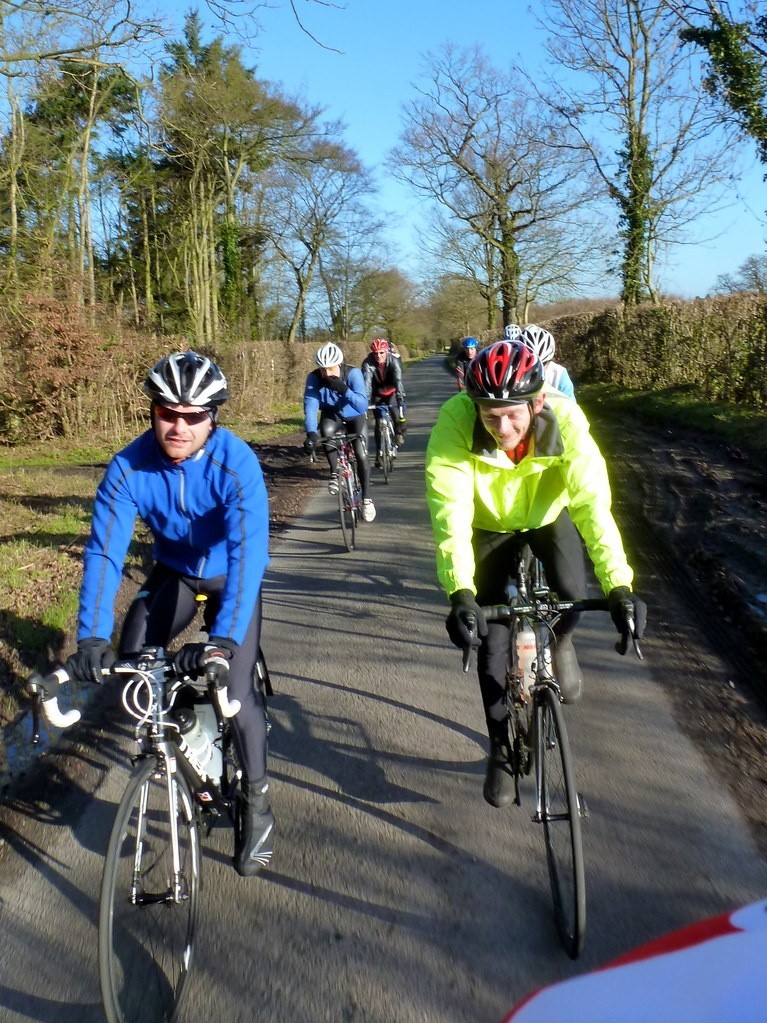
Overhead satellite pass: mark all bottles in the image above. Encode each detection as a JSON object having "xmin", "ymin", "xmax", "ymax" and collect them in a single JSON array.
[
  {"xmin": 516, "ymin": 617, "xmax": 538, "ymax": 696},
  {"xmin": 348, "ymin": 463, "xmax": 354, "ymax": 482},
  {"xmin": 172, "ymin": 707, "xmax": 213, "ymax": 767}
]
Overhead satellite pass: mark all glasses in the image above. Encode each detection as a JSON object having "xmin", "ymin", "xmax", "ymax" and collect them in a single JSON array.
[
  {"xmin": 374, "ymin": 352, "xmax": 385, "ymax": 355},
  {"xmin": 321, "ymin": 367, "xmax": 336, "ymax": 372},
  {"xmin": 152, "ymin": 398, "xmax": 213, "ymax": 424}
]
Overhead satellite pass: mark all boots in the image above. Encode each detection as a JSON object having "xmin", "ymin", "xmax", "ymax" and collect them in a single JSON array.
[
  {"xmin": 549, "ymin": 628, "xmax": 585, "ymax": 703},
  {"xmin": 482, "ymin": 731, "xmax": 515, "ymax": 808},
  {"xmin": 235, "ymin": 772, "xmax": 278, "ymax": 877}
]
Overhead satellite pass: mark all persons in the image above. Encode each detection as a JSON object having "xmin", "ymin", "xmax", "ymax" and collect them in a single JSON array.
[
  {"xmin": 456, "ymin": 336, "xmax": 485, "ymax": 392},
  {"xmin": 503, "ymin": 323, "xmax": 522, "ymax": 340},
  {"xmin": 60, "ymin": 350, "xmax": 276, "ymax": 876},
  {"xmin": 517, "ymin": 323, "xmax": 576, "ymax": 403},
  {"xmin": 303, "ymin": 342, "xmax": 377, "ymax": 522},
  {"xmin": 361, "ymin": 339, "xmax": 408, "ymax": 467},
  {"xmin": 424, "ymin": 342, "xmax": 649, "ymax": 809}
]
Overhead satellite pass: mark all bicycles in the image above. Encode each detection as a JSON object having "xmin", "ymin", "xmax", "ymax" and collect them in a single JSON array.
[
  {"xmin": 365, "ymin": 397, "xmax": 406, "ymax": 485},
  {"xmin": 309, "ymin": 432, "xmax": 368, "ymax": 553},
  {"xmin": 456, "ymin": 547, "xmax": 646, "ymax": 962},
  {"xmin": 26, "ymin": 597, "xmax": 275, "ymax": 1023}
]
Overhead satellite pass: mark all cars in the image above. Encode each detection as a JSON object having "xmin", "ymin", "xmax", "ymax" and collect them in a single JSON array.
[{"xmin": 500, "ymin": 899, "xmax": 766, "ymax": 1023}]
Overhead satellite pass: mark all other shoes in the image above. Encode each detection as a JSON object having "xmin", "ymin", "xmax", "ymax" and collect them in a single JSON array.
[
  {"xmin": 397, "ymin": 433, "xmax": 404, "ymax": 446},
  {"xmin": 375, "ymin": 456, "xmax": 382, "ymax": 466}
]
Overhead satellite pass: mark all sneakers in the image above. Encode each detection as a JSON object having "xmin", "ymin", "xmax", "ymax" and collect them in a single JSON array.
[
  {"xmin": 361, "ymin": 499, "xmax": 376, "ymax": 522},
  {"xmin": 328, "ymin": 473, "xmax": 341, "ymax": 494}
]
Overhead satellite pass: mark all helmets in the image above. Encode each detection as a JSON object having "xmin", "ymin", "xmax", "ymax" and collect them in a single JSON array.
[
  {"xmin": 465, "ymin": 339, "xmax": 544, "ymax": 406},
  {"xmin": 503, "ymin": 323, "xmax": 520, "ymax": 340},
  {"xmin": 463, "ymin": 337, "xmax": 478, "ymax": 348},
  {"xmin": 515, "ymin": 324, "xmax": 556, "ymax": 362},
  {"xmin": 315, "ymin": 341, "xmax": 343, "ymax": 368},
  {"xmin": 368, "ymin": 338, "xmax": 387, "ymax": 351},
  {"xmin": 144, "ymin": 350, "xmax": 228, "ymax": 406}
]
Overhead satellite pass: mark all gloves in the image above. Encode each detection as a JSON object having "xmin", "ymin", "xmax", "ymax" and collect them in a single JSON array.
[
  {"xmin": 322, "ymin": 375, "xmax": 349, "ymax": 396},
  {"xmin": 396, "ymin": 397, "xmax": 404, "ymax": 409},
  {"xmin": 66, "ymin": 638, "xmax": 114, "ymax": 685},
  {"xmin": 303, "ymin": 431, "xmax": 318, "ymax": 456},
  {"xmin": 173, "ymin": 641, "xmax": 237, "ymax": 682},
  {"xmin": 445, "ymin": 588, "xmax": 488, "ymax": 651},
  {"xmin": 609, "ymin": 583, "xmax": 647, "ymax": 638}
]
